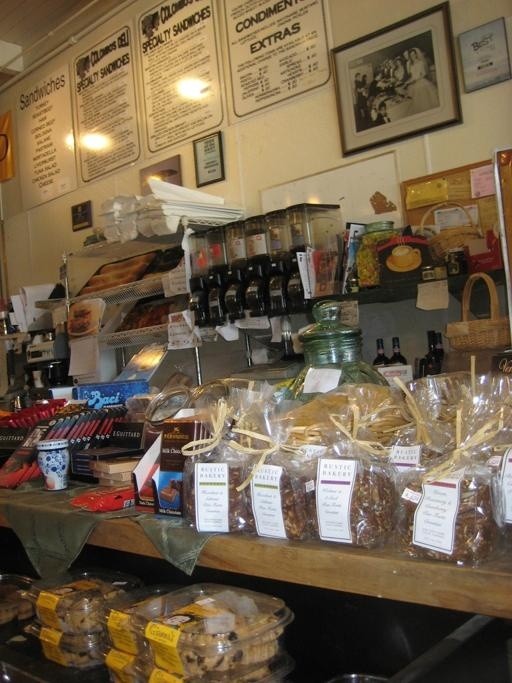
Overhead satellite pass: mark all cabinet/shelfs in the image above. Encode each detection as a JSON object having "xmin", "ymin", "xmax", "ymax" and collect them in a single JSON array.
[{"xmin": 63, "ymin": 216, "xmax": 254, "ymax": 389}]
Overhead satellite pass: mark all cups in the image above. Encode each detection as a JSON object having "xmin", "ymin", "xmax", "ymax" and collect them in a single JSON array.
[{"xmin": 392, "ymin": 244, "xmax": 421, "ymax": 265}]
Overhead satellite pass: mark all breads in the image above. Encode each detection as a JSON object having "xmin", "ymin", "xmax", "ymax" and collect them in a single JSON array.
[
  {"xmin": 0, "ymin": 574, "xmax": 282, "ymax": 682},
  {"xmin": 83, "ymin": 275, "xmax": 134, "ymax": 293},
  {"xmin": 88, "ymin": 264, "xmax": 146, "ymax": 284},
  {"xmin": 99, "ymin": 252, "xmax": 155, "ymax": 274},
  {"xmin": 178, "ymin": 376, "xmax": 512, "ymax": 573}
]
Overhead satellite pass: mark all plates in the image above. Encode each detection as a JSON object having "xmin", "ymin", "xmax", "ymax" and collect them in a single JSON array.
[{"xmin": 385, "ymin": 258, "xmax": 422, "ymax": 273}]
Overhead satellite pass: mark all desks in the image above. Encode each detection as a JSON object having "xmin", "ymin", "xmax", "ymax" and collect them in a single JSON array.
[{"xmin": 1, "ymin": 483, "xmax": 511, "ymax": 681}]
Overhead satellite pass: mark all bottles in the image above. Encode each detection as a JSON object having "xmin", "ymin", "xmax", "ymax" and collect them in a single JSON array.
[
  {"xmin": 355, "ymin": 220, "xmax": 402, "ymax": 289},
  {"xmin": 281, "ymin": 300, "xmax": 446, "ymax": 405}
]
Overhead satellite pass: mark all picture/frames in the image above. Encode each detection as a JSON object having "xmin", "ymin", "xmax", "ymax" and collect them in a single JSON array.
[
  {"xmin": 328, "ymin": 1, "xmax": 464, "ymax": 159},
  {"xmin": 192, "ymin": 130, "xmax": 225, "ymax": 187},
  {"xmin": 71, "ymin": 200, "xmax": 93, "ymax": 232}
]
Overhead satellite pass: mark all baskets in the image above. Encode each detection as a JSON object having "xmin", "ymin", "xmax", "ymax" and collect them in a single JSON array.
[
  {"xmin": 419, "ymin": 200, "xmax": 485, "ymax": 262},
  {"xmin": 445, "ymin": 270, "xmax": 512, "ymax": 350}
]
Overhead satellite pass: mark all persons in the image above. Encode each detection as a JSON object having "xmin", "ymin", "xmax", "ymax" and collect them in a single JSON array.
[{"xmin": 352, "ymin": 46, "xmax": 436, "ymax": 128}]
